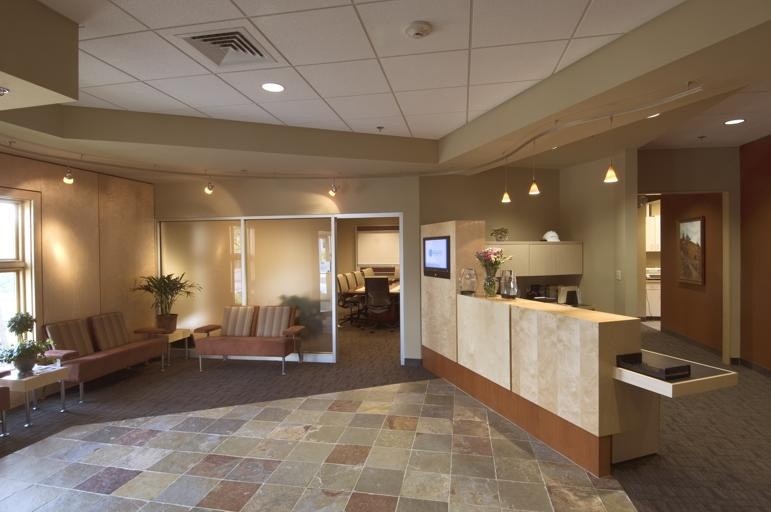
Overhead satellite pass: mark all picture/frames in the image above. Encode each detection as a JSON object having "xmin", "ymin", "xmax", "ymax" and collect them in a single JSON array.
[{"xmin": 676, "ymin": 215, "xmax": 705, "ymax": 286}]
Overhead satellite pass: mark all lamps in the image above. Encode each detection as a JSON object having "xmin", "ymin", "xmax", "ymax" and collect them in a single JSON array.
[
  {"xmin": 501, "ymin": 158, "xmax": 512, "ymax": 203},
  {"xmin": 62, "ymin": 159, "xmax": 73, "ymax": 184},
  {"xmin": 603, "ymin": 116, "xmax": 619, "ymax": 183},
  {"xmin": 203, "ymin": 176, "xmax": 215, "ymax": 195},
  {"xmin": 528, "ymin": 140, "xmax": 541, "ymax": 195},
  {"xmin": 329, "ymin": 177, "xmax": 337, "ymax": 196}
]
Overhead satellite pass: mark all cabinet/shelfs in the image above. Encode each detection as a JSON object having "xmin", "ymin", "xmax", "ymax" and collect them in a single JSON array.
[{"xmin": 486, "ymin": 240, "xmax": 583, "ymax": 278}]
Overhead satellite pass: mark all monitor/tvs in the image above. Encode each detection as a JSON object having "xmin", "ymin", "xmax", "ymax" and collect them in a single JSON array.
[{"xmin": 423, "ymin": 235, "xmax": 450, "ymax": 279}]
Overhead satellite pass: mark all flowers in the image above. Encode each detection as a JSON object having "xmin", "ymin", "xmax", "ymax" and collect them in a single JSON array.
[{"xmin": 474, "ymin": 249, "xmax": 513, "ymax": 296}]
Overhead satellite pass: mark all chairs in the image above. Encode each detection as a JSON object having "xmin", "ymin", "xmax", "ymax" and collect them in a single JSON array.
[
  {"xmin": 0, "ymin": 371, "xmax": 11, "ymax": 437},
  {"xmin": 337, "ymin": 266, "xmax": 400, "ymax": 333}
]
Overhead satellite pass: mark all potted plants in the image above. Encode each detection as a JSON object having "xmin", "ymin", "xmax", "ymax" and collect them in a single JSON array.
[
  {"xmin": 490, "ymin": 227, "xmax": 509, "ymax": 241},
  {"xmin": 0, "ymin": 312, "xmax": 57, "ymax": 378},
  {"xmin": 129, "ymin": 272, "xmax": 203, "ymax": 334}
]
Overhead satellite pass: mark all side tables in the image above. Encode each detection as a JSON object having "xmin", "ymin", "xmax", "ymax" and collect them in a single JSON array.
[
  {"xmin": 0, "ymin": 365, "xmax": 72, "ymax": 427},
  {"xmin": 152, "ymin": 329, "xmax": 191, "ymax": 366}
]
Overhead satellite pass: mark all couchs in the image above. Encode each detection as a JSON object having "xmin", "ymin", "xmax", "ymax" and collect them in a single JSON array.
[
  {"xmin": 39, "ymin": 311, "xmax": 168, "ymax": 404},
  {"xmin": 192, "ymin": 305, "xmax": 308, "ymax": 376}
]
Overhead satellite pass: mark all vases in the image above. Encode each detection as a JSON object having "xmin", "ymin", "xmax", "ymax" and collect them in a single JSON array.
[{"xmin": 482, "ymin": 275, "xmax": 499, "ymax": 297}]
